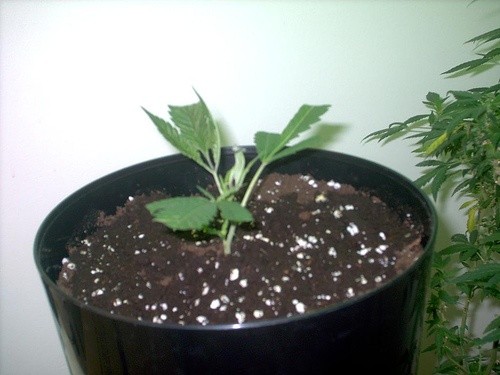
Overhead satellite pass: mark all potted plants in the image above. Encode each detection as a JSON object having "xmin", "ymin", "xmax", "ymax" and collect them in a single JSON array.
[{"xmin": 33, "ymin": 91, "xmax": 439, "ymax": 373}]
